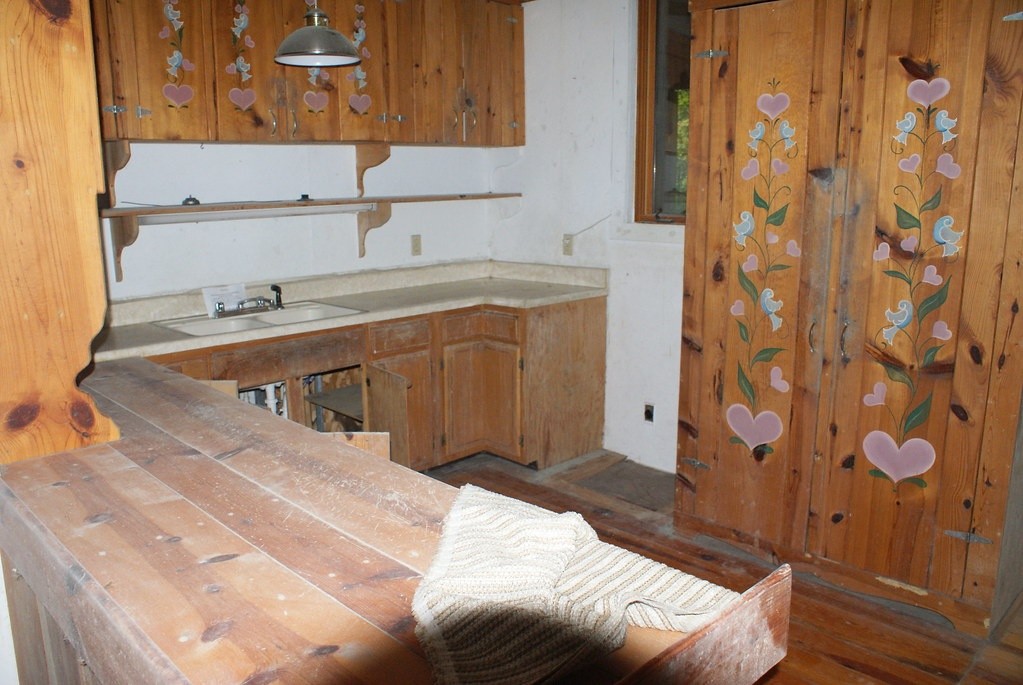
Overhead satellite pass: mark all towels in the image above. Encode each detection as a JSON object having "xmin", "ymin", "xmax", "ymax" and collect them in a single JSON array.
[{"xmin": 408, "ymin": 481, "xmax": 743, "ymax": 685}]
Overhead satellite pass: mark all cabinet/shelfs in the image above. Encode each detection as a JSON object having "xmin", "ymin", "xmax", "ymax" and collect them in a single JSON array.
[
  {"xmin": 488, "ymin": 296, "xmax": 609, "ymax": 471},
  {"xmin": 416, "ymin": 1, "xmax": 524, "ymax": 143},
  {"xmin": 206, "ymin": 324, "xmax": 411, "ymax": 472},
  {"xmin": 676, "ymin": 3, "xmax": 1019, "ymax": 636},
  {"xmin": 217, "ymin": 1, "xmax": 338, "ymax": 141},
  {"xmin": 371, "ymin": 303, "xmax": 482, "ymax": 473},
  {"xmin": 96, "ymin": 1, "xmax": 213, "ymax": 142},
  {"xmin": 339, "ymin": 4, "xmax": 411, "ymax": 140}
]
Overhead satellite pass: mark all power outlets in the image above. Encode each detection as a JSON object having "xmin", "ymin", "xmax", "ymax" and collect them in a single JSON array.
[
  {"xmin": 644, "ymin": 404, "xmax": 655, "ymax": 422},
  {"xmin": 563, "ymin": 233, "xmax": 573, "ymax": 256}
]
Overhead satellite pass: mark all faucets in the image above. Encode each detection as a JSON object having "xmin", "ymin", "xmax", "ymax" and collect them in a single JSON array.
[{"xmin": 237, "ymin": 296, "xmax": 275, "ymax": 311}]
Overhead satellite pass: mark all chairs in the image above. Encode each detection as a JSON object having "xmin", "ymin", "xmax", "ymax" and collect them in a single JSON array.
[{"xmin": 272, "ymin": 2, "xmax": 361, "ymax": 68}]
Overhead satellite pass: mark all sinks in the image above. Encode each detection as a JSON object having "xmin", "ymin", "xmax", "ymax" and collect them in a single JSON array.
[
  {"xmin": 253, "ymin": 299, "xmax": 371, "ymax": 326},
  {"xmin": 150, "ymin": 314, "xmax": 271, "ymax": 338}
]
